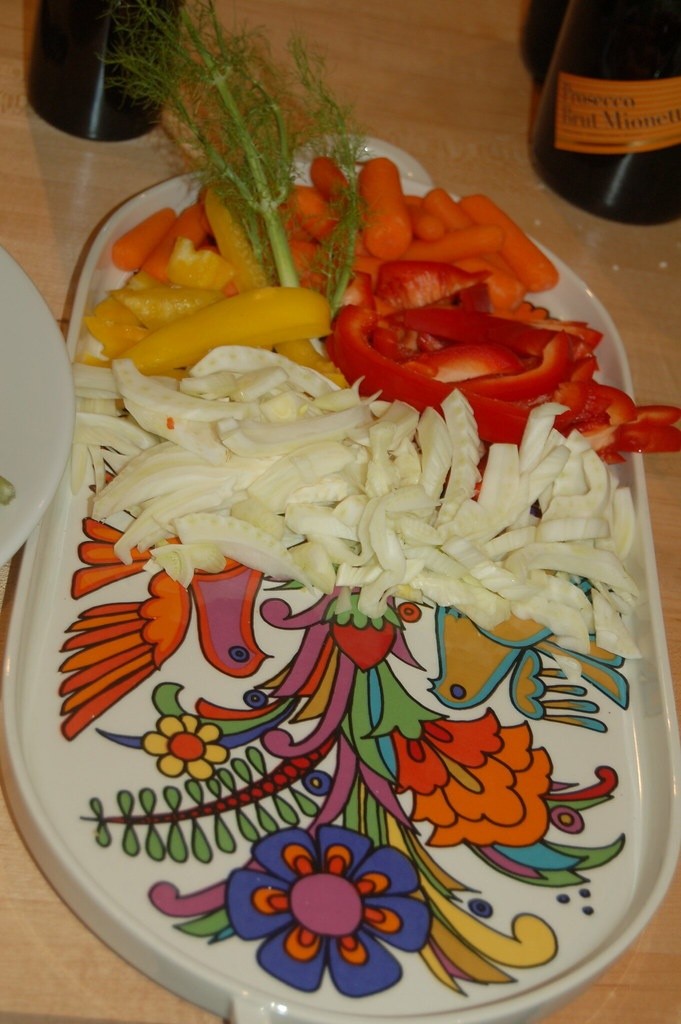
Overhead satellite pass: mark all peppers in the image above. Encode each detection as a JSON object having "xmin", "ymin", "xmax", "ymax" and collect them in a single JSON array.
[{"xmin": 71, "ymin": 183, "xmax": 680, "ymax": 467}]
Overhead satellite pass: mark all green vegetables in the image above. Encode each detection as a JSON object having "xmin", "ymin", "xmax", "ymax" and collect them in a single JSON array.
[{"xmin": 98, "ymin": 0, "xmax": 380, "ymax": 307}]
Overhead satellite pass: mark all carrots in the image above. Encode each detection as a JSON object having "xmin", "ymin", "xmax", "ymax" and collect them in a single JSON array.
[{"xmin": 111, "ymin": 155, "xmax": 558, "ymax": 314}]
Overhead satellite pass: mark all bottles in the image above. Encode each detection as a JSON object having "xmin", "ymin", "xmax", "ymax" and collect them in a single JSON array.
[
  {"xmin": 24, "ymin": 0, "xmax": 185, "ymax": 144},
  {"xmin": 517, "ymin": 0, "xmax": 681, "ymax": 227}
]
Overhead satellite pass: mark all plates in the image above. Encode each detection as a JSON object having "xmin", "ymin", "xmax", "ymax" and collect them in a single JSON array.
[
  {"xmin": 0, "ymin": 133, "xmax": 679, "ymax": 1024},
  {"xmin": 0, "ymin": 245, "xmax": 76, "ymax": 567}
]
